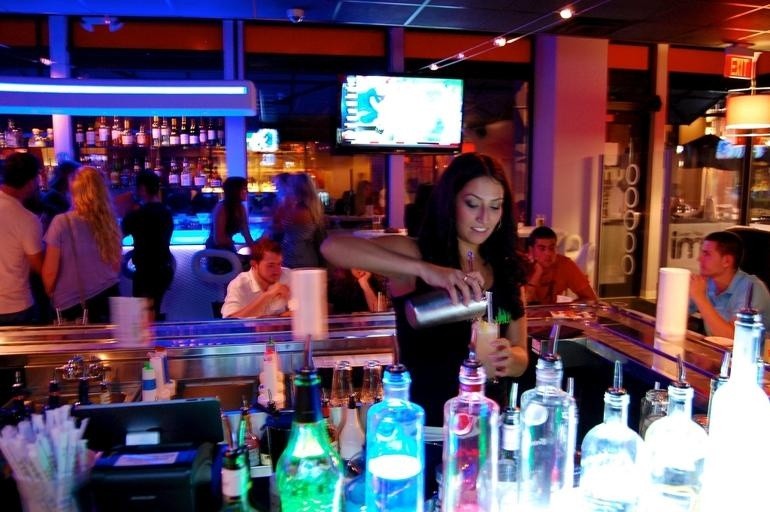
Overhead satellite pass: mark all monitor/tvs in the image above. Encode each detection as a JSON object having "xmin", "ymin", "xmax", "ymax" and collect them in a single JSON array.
[
  {"xmin": 245, "ymin": 128, "xmax": 279, "ymax": 153},
  {"xmin": 699, "ymin": 134, "xmax": 769, "ymax": 168},
  {"xmin": 328, "ymin": 69, "xmax": 467, "ymax": 156}
]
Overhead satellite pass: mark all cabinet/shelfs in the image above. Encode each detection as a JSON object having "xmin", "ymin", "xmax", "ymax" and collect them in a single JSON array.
[{"xmin": 212, "ymin": 141, "xmax": 309, "ymax": 186}]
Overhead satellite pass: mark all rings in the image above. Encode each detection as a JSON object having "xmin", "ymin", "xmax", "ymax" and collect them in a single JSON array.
[{"xmin": 462, "ymin": 273, "xmax": 470, "ymax": 285}]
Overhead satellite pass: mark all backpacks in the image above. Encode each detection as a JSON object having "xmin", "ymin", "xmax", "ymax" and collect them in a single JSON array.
[{"xmin": 48, "ymin": 212, "xmax": 89, "ymax": 326}]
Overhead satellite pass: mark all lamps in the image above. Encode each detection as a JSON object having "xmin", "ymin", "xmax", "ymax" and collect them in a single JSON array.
[{"xmin": 723, "ymin": 51, "xmax": 770, "ymax": 139}]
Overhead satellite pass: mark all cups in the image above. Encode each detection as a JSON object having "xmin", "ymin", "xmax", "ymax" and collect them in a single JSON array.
[{"xmin": 475, "ymin": 319, "xmax": 500, "ymax": 381}]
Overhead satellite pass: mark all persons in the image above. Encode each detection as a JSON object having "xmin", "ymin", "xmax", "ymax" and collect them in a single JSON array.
[
  {"xmin": 221, "ymin": 238, "xmax": 298, "ymax": 319},
  {"xmin": 270, "ymin": 171, "xmax": 327, "ymax": 268},
  {"xmin": 523, "ymin": 226, "xmax": 597, "ymax": 303},
  {"xmin": 320, "ymin": 151, "xmax": 528, "ymax": 430},
  {"xmin": 116, "ymin": 169, "xmax": 177, "ymax": 315},
  {"xmin": 354, "ymin": 181, "xmax": 371, "ymax": 215},
  {"xmin": 513, "ymin": 199, "xmax": 527, "ymax": 227},
  {"xmin": 0, "ymin": 153, "xmax": 43, "ymax": 326},
  {"xmin": 688, "ymin": 232, "xmax": 770, "ymax": 340},
  {"xmin": 275, "ymin": 173, "xmax": 292, "ymax": 200},
  {"xmin": 41, "ymin": 159, "xmax": 83, "ymax": 214},
  {"xmin": 41, "ymin": 165, "xmax": 123, "ymax": 326},
  {"xmin": 206, "ymin": 177, "xmax": 256, "ymax": 274},
  {"xmin": 371, "ymin": 187, "xmax": 387, "ymax": 216}
]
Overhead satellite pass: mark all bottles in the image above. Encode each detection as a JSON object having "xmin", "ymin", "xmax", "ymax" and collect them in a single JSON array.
[
  {"xmin": 141, "ymin": 362, "xmax": 156, "ymax": 402},
  {"xmin": 646, "ymin": 383, "xmax": 708, "ymax": 512},
  {"xmin": 443, "ymin": 359, "xmax": 498, "ymax": 512},
  {"xmin": 199, "ymin": 120, "xmax": 208, "ymax": 144},
  {"xmin": 340, "ymin": 397, "xmax": 365, "ymax": 464},
  {"xmin": 216, "ymin": 119, "xmax": 224, "ymax": 146},
  {"xmin": 705, "ymin": 310, "xmax": 770, "ymax": 511},
  {"xmin": 70, "ymin": 378, "xmax": 93, "ymax": 423},
  {"xmin": 180, "ymin": 118, "xmax": 189, "ymax": 144},
  {"xmin": 194, "ymin": 159, "xmax": 207, "ymax": 189},
  {"xmin": 42, "ymin": 381, "xmax": 65, "ymax": 422},
  {"xmin": 189, "ymin": 120, "xmax": 199, "ymax": 145},
  {"xmin": 362, "ymin": 359, "xmax": 383, "ymax": 412},
  {"xmin": 211, "ymin": 166, "xmax": 221, "ymax": 187},
  {"xmin": 219, "ymin": 449, "xmax": 257, "ymax": 512},
  {"xmin": 518, "ymin": 355, "xmax": 577, "ymax": 510},
  {"xmin": 170, "ymin": 119, "xmax": 180, "ymax": 145},
  {"xmin": 220, "ymin": 414, "xmax": 232, "ymax": 448},
  {"xmin": 580, "ymin": 388, "xmax": 646, "ymax": 512},
  {"xmin": 28, "ymin": 129, "xmax": 45, "ymax": 147},
  {"xmin": 110, "ymin": 156, "xmax": 121, "ymax": 188},
  {"xmin": 501, "ymin": 410, "xmax": 520, "ymax": 456},
  {"xmin": 76, "ymin": 125, "xmax": 84, "ymax": 146},
  {"xmin": 329, "ymin": 361, "xmax": 358, "ymax": 426},
  {"xmin": 136, "ymin": 126, "xmax": 150, "ymax": 145},
  {"xmin": 99, "ymin": 118, "xmax": 111, "ymax": 146},
  {"xmin": 112, "ymin": 119, "xmax": 123, "ymax": 146},
  {"xmin": 122, "ymin": 121, "xmax": 133, "ymax": 145},
  {"xmin": 86, "ymin": 128, "xmax": 96, "ymax": 147},
  {"xmin": 365, "ymin": 366, "xmax": 424, "ymax": 512},
  {"xmin": 161, "ymin": 121, "xmax": 171, "ymax": 146},
  {"xmin": 208, "ymin": 121, "xmax": 216, "ymax": 145},
  {"xmin": 154, "ymin": 159, "xmax": 163, "ymax": 185},
  {"xmin": 236, "ymin": 407, "xmax": 253, "ymax": 450},
  {"xmin": 277, "ymin": 369, "xmax": 342, "ymax": 512},
  {"xmin": 152, "ymin": 118, "xmax": 161, "ymax": 145},
  {"xmin": 5, "ymin": 120, "xmax": 22, "ymax": 147},
  {"xmin": 168, "ymin": 161, "xmax": 180, "ymax": 187},
  {"xmin": 181, "ymin": 157, "xmax": 192, "ymax": 188},
  {"xmin": 2, "ymin": 385, "xmax": 33, "ymax": 423},
  {"xmin": 120, "ymin": 160, "xmax": 132, "ymax": 187}
]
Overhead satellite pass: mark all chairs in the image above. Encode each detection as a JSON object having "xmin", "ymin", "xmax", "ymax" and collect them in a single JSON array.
[
  {"xmin": 190, "ymin": 250, "xmax": 241, "ymax": 317},
  {"xmin": 120, "ymin": 248, "xmax": 177, "ymax": 321}
]
